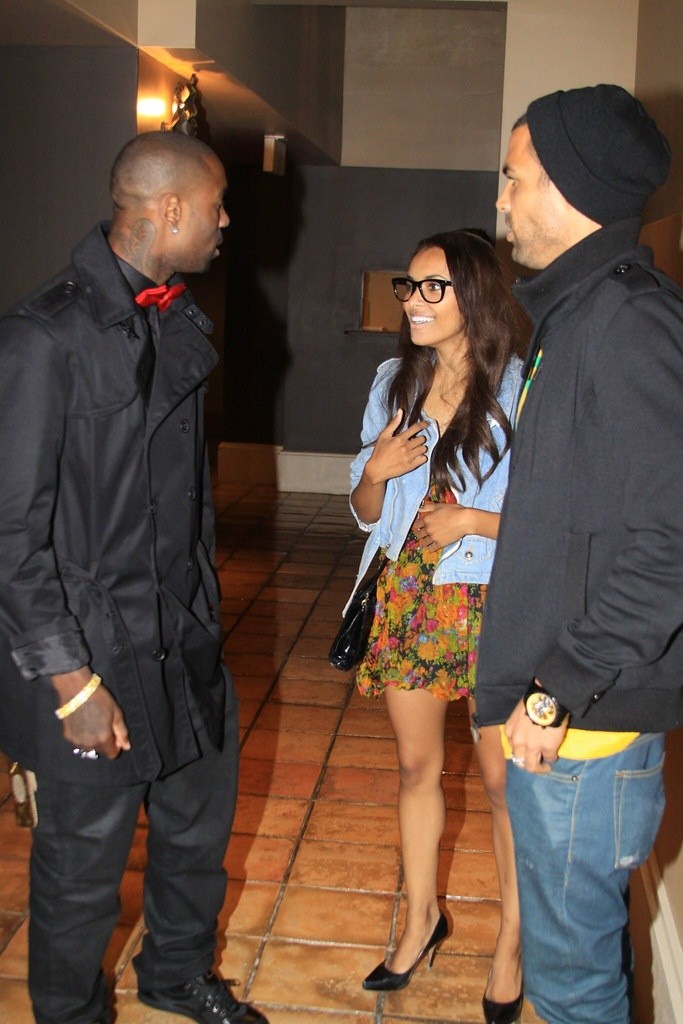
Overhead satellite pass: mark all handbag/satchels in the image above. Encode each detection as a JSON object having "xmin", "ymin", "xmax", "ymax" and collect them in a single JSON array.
[{"xmin": 328, "ymin": 577, "xmax": 378, "ymax": 672}]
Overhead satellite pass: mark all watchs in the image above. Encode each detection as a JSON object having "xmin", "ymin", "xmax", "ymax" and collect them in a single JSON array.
[{"xmin": 523, "ymin": 677, "xmax": 570, "ymax": 728}]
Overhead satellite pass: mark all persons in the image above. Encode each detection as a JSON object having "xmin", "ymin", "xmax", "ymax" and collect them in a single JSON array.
[
  {"xmin": 327, "ymin": 227, "xmax": 528, "ymax": 1023},
  {"xmin": 472, "ymin": 83, "xmax": 683, "ymax": 1024},
  {"xmin": 0, "ymin": 134, "xmax": 271, "ymax": 1024}
]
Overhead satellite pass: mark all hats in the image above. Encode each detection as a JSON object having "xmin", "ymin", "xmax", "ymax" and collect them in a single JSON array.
[{"xmin": 527, "ymin": 84, "xmax": 671, "ymax": 226}]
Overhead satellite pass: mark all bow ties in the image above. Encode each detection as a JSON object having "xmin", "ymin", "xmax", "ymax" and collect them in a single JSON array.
[{"xmin": 135, "ymin": 283, "xmax": 187, "ymax": 313}]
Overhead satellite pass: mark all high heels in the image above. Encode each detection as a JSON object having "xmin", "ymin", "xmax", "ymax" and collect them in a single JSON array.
[
  {"xmin": 482, "ymin": 966, "xmax": 523, "ymax": 1024},
  {"xmin": 363, "ymin": 910, "xmax": 448, "ymax": 991}
]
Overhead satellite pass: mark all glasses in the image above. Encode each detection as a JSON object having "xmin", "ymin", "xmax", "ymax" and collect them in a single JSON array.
[{"xmin": 392, "ymin": 277, "xmax": 452, "ymax": 304}]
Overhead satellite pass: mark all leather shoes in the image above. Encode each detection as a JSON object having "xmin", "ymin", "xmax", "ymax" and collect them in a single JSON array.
[{"xmin": 137, "ymin": 970, "xmax": 269, "ymax": 1024}]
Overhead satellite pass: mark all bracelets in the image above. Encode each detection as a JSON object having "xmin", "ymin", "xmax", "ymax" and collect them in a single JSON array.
[{"xmin": 53, "ymin": 672, "xmax": 104, "ymax": 720}]
